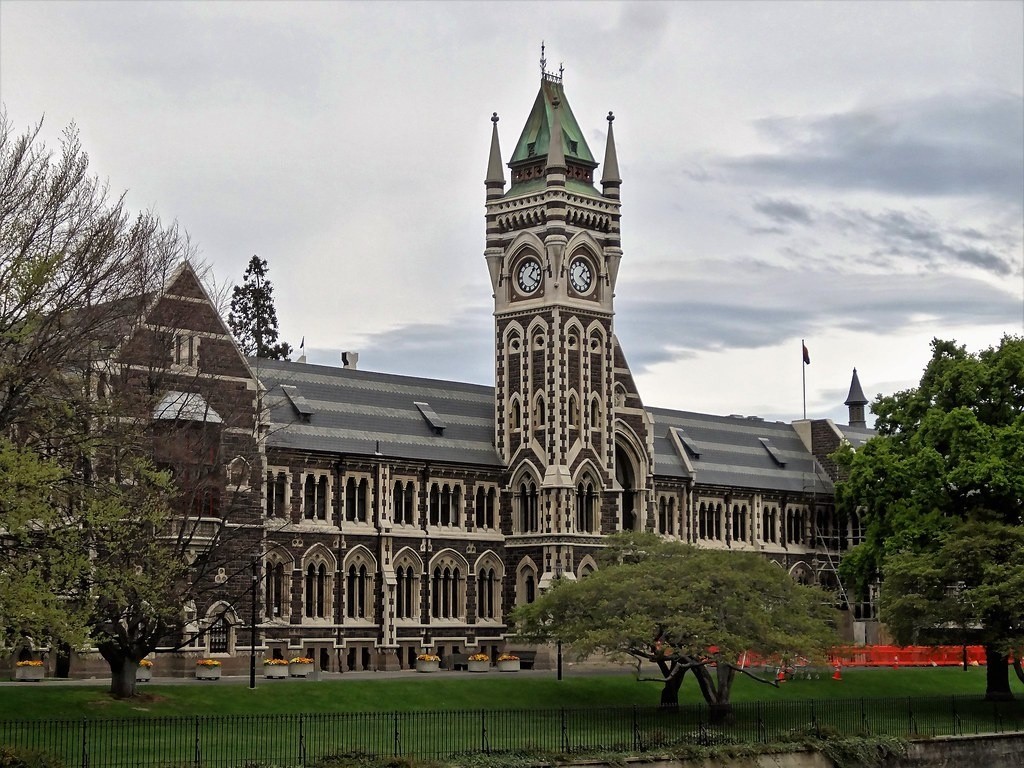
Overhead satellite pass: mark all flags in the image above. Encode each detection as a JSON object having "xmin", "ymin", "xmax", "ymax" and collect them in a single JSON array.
[{"xmin": 803, "ymin": 345, "xmax": 810, "ymax": 363}]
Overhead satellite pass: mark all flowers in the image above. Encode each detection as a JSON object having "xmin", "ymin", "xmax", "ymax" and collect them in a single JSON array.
[
  {"xmin": 468, "ymin": 653, "xmax": 490, "ymax": 662},
  {"xmin": 196, "ymin": 659, "xmax": 222, "ymax": 670},
  {"xmin": 498, "ymin": 655, "xmax": 520, "ymax": 661},
  {"xmin": 16, "ymin": 659, "xmax": 43, "ymax": 668},
  {"xmin": 416, "ymin": 654, "xmax": 441, "ymax": 662},
  {"xmin": 264, "ymin": 658, "xmax": 289, "ymax": 665},
  {"xmin": 137, "ymin": 659, "xmax": 153, "ymax": 671},
  {"xmin": 290, "ymin": 657, "xmax": 314, "ymax": 664}
]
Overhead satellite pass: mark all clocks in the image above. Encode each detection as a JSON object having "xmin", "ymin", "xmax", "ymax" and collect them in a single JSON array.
[
  {"xmin": 517, "ymin": 260, "xmax": 541, "ymax": 293},
  {"xmin": 570, "ymin": 260, "xmax": 591, "ymax": 292}
]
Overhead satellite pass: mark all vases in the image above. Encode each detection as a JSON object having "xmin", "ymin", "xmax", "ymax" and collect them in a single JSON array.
[
  {"xmin": 16, "ymin": 667, "xmax": 44, "ymax": 682},
  {"xmin": 196, "ymin": 667, "xmax": 221, "ymax": 680},
  {"xmin": 136, "ymin": 668, "xmax": 151, "ymax": 681},
  {"xmin": 266, "ymin": 665, "xmax": 288, "ymax": 679},
  {"xmin": 468, "ymin": 661, "xmax": 489, "ymax": 671},
  {"xmin": 417, "ymin": 660, "xmax": 439, "ymax": 672},
  {"xmin": 290, "ymin": 663, "xmax": 314, "ymax": 678},
  {"xmin": 498, "ymin": 660, "xmax": 520, "ymax": 671}
]
